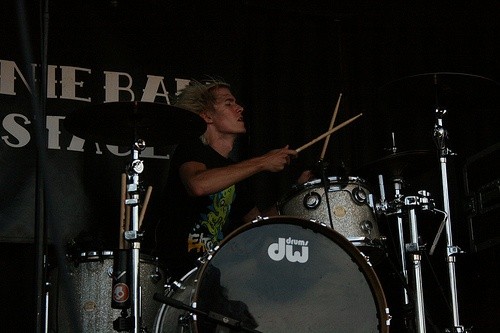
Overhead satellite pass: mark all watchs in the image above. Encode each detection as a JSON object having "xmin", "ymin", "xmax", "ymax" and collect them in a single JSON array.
[{"xmin": 291, "ymin": 184, "xmax": 297, "ymax": 190}]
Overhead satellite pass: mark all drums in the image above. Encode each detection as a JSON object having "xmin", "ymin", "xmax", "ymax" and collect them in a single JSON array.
[
  {"xmin": 45, "ymin": 249, "xmax": 175, "ymax": 333},
  {"xmin": 276, "ymin": 175, "xmax": 389, "ymax": 252},
  {"xmin": 156, "ymin": 215, "xmax": 392, "ymax": 333}
]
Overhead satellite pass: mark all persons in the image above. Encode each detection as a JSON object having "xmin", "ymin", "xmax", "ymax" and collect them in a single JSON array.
[{"xmin": 151, "ymin": 78, "xmax": 314, "ymax": 282}]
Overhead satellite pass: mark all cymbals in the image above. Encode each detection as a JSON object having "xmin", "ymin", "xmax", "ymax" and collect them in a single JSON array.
[
  {"xmin": 372, "ymin": 149, "xmax": 433, "ymax": 168},
  {"xmin": 64, "ymin": 101, "xmax": 208, "ymax": 148},
  {"xmin": 378, "ymin": 72, "xmax": 496, "ymax": 105}
]
